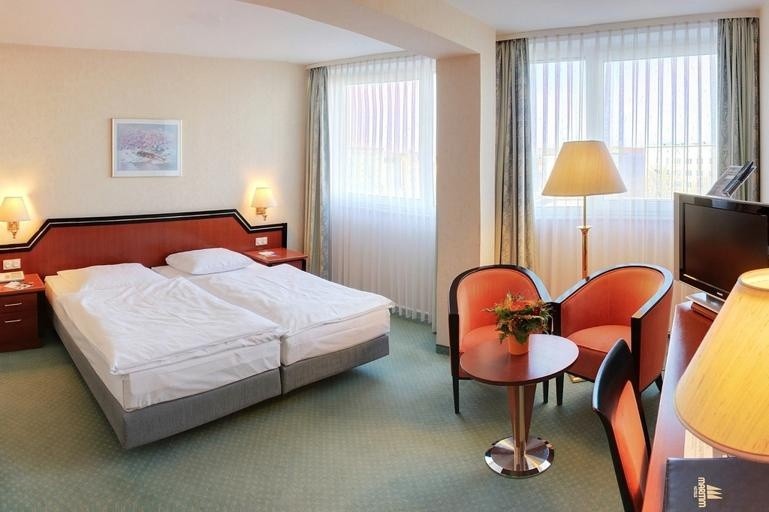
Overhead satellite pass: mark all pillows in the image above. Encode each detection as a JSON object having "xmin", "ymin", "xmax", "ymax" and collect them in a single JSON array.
[
  {"xmin": 56, "ymin": 262, "xmax": 167, "ymax": 292},
  {"xmin": 165, "ymin": 247, "xmax": 255, "ymax": 275}
]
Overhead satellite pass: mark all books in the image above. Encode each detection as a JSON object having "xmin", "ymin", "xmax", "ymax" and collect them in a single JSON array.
[{"xmin": 661, "ymin": 456, "xmax": 769, "ymax": 512}]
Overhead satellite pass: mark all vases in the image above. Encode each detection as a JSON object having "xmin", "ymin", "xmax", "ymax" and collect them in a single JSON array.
[{"xmin": 507, "ymin": 334, "xmax": 529, "ymax": 355}]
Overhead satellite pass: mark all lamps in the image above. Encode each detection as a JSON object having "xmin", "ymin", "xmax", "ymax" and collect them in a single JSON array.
[
  {"xmin": 0, "ymin": 197, "xmax": 31, "ymax": 239},
  {"xmin": 542, "ymin": 140, "xmax": 628, "ymax": 280},
  {"xmin": 673, "ymin": 268, "xmax": 769, "ymax": 464},
  {"xmin": 251, "ymin": 184, "xmax": 277, "ymax": 221}
]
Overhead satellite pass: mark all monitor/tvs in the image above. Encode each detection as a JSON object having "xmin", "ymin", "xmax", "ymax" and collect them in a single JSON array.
[{"xmin": 672, "ymin": 191, "xmax": 769, "ymax": 314}]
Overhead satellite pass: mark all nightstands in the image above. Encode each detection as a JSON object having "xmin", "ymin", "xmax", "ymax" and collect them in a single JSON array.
[
  {"xmin": 0, "ymin": 273, "xmax": 46, "ymax": 354},
  {"xmin": 243, "ymin": 247, "xmax": 308, "ymax": 272}
]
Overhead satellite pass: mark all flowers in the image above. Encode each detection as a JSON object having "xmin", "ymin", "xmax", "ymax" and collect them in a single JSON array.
[{"xmin": 479, "ymin": 289, "xmax": 553, "ymax": 346}]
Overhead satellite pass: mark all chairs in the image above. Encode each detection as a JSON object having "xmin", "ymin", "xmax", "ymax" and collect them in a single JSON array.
[
  {"xmin": 591, "ymin": 336, "xmax": 652, "ymax": 512},
  {"xmin": 554, "ymin": 262, "xmax": 674, "ymax": 407},
  {"xmin": 447, "ymin": 264, "xmax": 553, "ymax": 414}
]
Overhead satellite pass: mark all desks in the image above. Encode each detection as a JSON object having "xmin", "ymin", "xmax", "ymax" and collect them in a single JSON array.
[{"xmin": 641, "ymin": 299, "xmax": 737, "ymax": 512}]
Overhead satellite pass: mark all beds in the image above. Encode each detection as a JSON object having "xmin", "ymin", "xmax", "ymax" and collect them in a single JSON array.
[
  {"xmin": 151, "ymin": 264, "xmax": 396, "ymax": 397},
  {"xmin": 43, "ymin": 262, "xmax": 282, "ymax": 452}
]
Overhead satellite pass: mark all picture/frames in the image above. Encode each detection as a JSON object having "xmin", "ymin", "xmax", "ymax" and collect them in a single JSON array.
[{"xmin": 111, "ymin": 118, "xmax": 183, "ymax": 178}]
